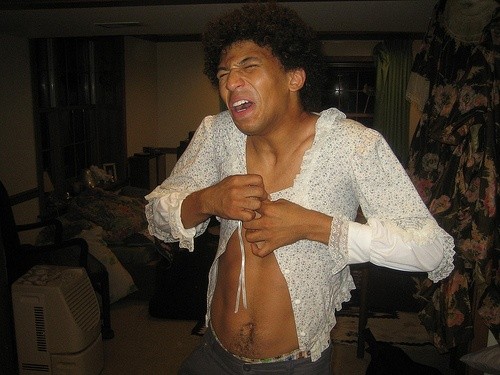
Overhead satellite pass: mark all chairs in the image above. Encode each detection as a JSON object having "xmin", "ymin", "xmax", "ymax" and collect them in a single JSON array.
[{"xmin": 1, "ymin": 161, "xmax": 159, "ymax": 341}]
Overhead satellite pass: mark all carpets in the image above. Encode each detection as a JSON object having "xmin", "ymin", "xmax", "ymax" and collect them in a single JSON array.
[{"xmin": 191, "ymin": 292, "xmax": 432, "ymax": 344}]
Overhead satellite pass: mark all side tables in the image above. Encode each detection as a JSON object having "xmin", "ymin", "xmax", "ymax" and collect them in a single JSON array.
[{"xmin": 91, "ymin": 166, "xmax": 149, "ymax": 196}]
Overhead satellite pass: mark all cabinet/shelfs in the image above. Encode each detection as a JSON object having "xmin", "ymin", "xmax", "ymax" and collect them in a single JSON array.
[{"xmin": 132, "ymin": 149, "xmax": 166, "ymax": 191}]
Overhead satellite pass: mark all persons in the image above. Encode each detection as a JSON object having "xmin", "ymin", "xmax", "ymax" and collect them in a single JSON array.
[{"xmin": 143, "ymin": 5, "xmax": 456, "ymax": 375}]
